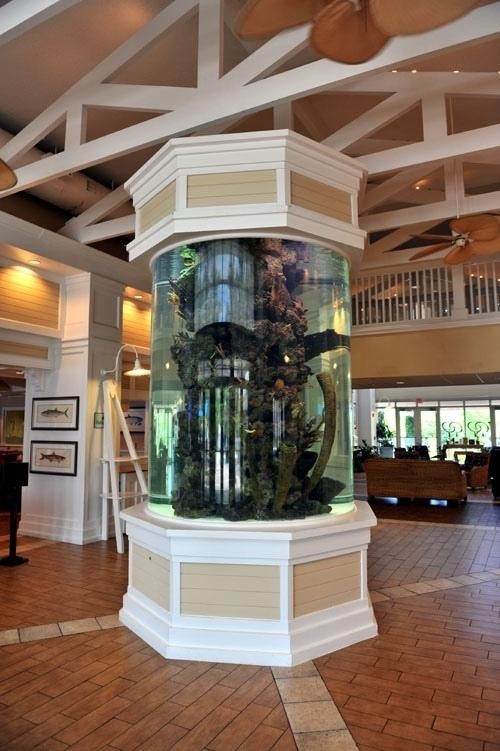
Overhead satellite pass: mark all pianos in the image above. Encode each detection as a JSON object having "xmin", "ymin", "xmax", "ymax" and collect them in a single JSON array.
[{"xmin": 0, "ymin": 453, "xmax": 28, "ymax": 565}]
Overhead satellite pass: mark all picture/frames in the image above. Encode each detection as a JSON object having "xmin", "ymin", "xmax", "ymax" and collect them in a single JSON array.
[
  {"xmin": 31, "ymin": 396, "xmax": 80, "ymax": 432},
  {"xmin": 120, "ymin": 406, "xmax": 146, "ymax": 434},
  {"xmin": 29, "ymin": 439, "xmax": 78, "ymax": 477},
  {"xmin": 1, "ymin": 405, "xmax": 24, "ymax": 449}
]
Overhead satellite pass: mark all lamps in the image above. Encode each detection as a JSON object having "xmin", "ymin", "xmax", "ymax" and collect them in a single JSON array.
[
  {"xmin": 407, "ymin": 213, "xmax": 500, "ymax": 269},
  {"xmin": 232, "ymin": 0, "xmax": 477, "ymax": 67},
  {"xmin": 101, "ymin": 343, "xmax": 152, "ymax": 381}
]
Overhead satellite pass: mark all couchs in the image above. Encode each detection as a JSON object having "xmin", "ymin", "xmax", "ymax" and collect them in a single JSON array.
[
  {"xmin": 468, "ymin": 451, "xmax": 491, "ymax": 490},
  {"xmin": 362, "ymin": 460, "xmax": 467, "ymax": 506}
]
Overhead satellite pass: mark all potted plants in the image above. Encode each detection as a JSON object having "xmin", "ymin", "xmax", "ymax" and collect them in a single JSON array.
[{"xmin": 378, "ymin": 440, "xmax": 395, "ymax": 458}]
